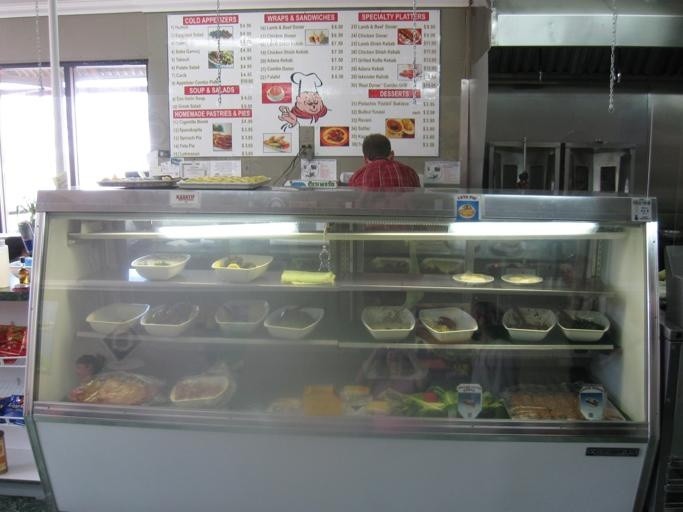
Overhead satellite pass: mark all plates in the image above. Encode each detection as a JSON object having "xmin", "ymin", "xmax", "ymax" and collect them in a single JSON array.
[
  {"xmin": 262, "ymin": 139, "xmax": 293, "ymax": 154},
  {"xmin": 452, "ymin": 273, "xmax": 493, "ymax": 285},
  {"xmin": 69, "ymin": 371, "xmax": 159, "ymax": 408},
  {"xmin": 266, "ymin": 87, "xmax": 284, "ymax": 102},
  {"xmin": 397, "ymin": 67, "xmax": 417, "ymax": 80},
  {"xmin": 320, "ymin": 128, "xmax": 348, "ymax": 146},
  {"xmin": 209, "ymin": 59, "xmax": 230, "ymax": 68},
  {"xmin": 168, "ymin": 375, "xmax": 229, "ymax": 410},
  {"xmin": 398, "ymin": 32, "xmax": 421, "ymax": 45},
  {"xmin": 500, "ymin": 274, "xmax": 543, "ymax": 285},
  {"xmin": 401, "ymin": 118, "xmax": 413, "ymax": 134},
  {"xmin": 212, "ymin": 131, "xmax": 231, "ymax": 150}
]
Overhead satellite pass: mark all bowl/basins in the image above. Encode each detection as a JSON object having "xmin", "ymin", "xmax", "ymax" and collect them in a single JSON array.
[
  {"xmin": 210, "ymin": 254, "xmax": 273, "ymax": 283},
  {"xmin": 417, "ymin": 305, "xmax": 477, "ymax": 343},
  {"xmin": 139, "ymin": 302, "xmax": 200, "ymax": 337},
  {"xmin": 262, "ymin": 305, "xmax": 324, "ymax": 339},
  {"xmin": 86, "ymin": 301, "xmax": 149, "ymax": 335},
  {"xmin": 130, "ymin": 252, "xmax": 191, "ymax": 282},
  {"xmin": 557, "ymin": 307, "xmax": 609, "ymax": 342},
  {"xmin": 361, "ymin": 305, "xmax": 414, "ymax": 341},
  {"xmin": 214, "ymin": 299, "xmax": 270, "ymax": 336},
  {"xmin": 501, "ymin": 307, "xmax": 556, "ymax": 342}
]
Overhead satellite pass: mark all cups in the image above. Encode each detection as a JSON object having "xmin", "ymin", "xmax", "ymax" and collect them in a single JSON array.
[
  {"xmin": 269, "ymin": 87, "xmax": 282, "ymax": 100},
  {"xmin": 386, "ymin": 119, "xmax": 402, "ymax": 134}
]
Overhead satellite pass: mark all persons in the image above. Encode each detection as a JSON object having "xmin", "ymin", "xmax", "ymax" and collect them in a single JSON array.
[{"xmin": 349, "ymin": 134, "xmax": 421, "ymax": 245}]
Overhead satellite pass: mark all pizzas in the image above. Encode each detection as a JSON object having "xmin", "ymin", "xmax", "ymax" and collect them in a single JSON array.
[{"xmin": 322, "ymin": 127, "xmax": 347, "ymax": 144}]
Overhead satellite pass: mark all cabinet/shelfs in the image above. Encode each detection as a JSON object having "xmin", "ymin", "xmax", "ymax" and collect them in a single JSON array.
[
  {"xmin": 0, "ymin": 284, "xmax": 42, "ymax": 488},
  {"xmin": 25, "ymin": 192, "xmax": 661, "ymax": 431}
]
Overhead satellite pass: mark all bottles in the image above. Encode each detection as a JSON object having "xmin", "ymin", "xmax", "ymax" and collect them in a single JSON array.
[
  {"xmin": 17, "ymin": 220, "xmax": 33, "ymax": 257},
  {"xmin": 0, "ymin": 239, "xmax": 10, "ymax": 289},
  {"xmin": 18, "ymin": 256, "xmax": 30, "ymax": 285},
  {"xmin": 318, "ymin": 245, "xmax": 332, "ymax": 273}
]
